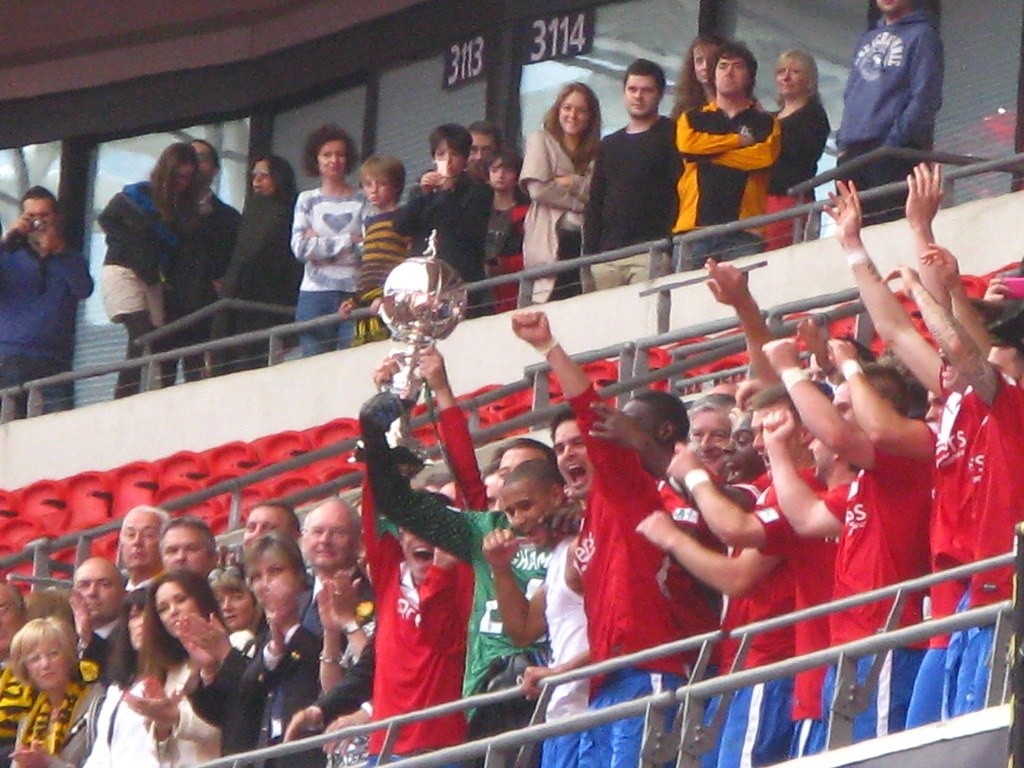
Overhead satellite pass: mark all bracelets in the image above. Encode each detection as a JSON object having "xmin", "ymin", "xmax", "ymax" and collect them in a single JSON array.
[
  {"xmin": 781, "ymin": 367, "xmax": 809, "ymax": 391},
  {"xmin": 537, "ymin": 336, "xmax": 559, "ymax": 356},
  {"xmin": 317, "ymin": 648, "xmax": 343, "ymax": 664},
  {"xmin": 684, "ymin": 468, "xmax": 709, "ymax": 491},
  {"xmin": 848, "ymin": 246, "xmax": 870, "ymax": 267},
  {"xmin": 341, "ymin": 620, "xmax": 360, "ymax": 633},
  {"xmin": 841, "ymin": 360, "xmax": 863, "ymax": 380}
]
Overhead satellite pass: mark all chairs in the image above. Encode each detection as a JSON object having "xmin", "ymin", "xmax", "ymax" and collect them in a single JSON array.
[{"xmin": 0, "ymin": 261, "xmax": 1024, "ymax": 573}]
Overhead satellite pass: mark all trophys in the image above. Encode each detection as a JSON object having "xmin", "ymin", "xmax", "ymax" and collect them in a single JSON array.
[{"xmin": 346, "ymin": 228, "xmax": 468, "ymax": 464}]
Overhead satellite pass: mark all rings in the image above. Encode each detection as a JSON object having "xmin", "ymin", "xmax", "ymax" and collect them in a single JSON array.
[{"xmin": 335, "ymin": 592, "xmax": 342, "ymax": 595}]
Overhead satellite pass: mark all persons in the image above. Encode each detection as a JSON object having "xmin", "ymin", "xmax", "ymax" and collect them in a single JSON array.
[
  {"xmin": 517, "ymin": 82, "xmax": 601, "ymax": 310},
  {"xmin": 0, "ymin": 503, "xmax": 376, "ymax": 768},
  {"xmin": 394, "ymin": 121, "xmax": 532, "ymax": 320},
  {"xmin": 671, "ymin": 34, "xmax": 783, "ymax": 274},
  {"xmin": 0, "ymin": 185, "xmax": 93, "ymax": 424},
  {"xmin": 203, "ymin": 124, "xmax": 413, "ymax": 379},
  {"xmin": 348, "ymin": 163, "xmax": 1024, "ymax": 768},
  {"xmin": 835, "ymin": 0, "xmax": 944, "ymax": 227},
  {"xmin": 94, "ymin": 139, "xmax": 242, "ymax": 400},
  {"xmin": 582, "ymin": 59, "xmax": 685, "ymax": 295},
  {"xmin": 764, "ymin": 50, "xmax": 831, "ymax": 252}
]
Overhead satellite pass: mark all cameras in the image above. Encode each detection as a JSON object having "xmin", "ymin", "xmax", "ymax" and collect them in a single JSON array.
[
  {"xmin": 1002, "ymin": 277, "xmax": 1024, "ymax": 299},
  {"xmin": 33, "ymin": 219, "xmax": 48, "ymax": 233}
]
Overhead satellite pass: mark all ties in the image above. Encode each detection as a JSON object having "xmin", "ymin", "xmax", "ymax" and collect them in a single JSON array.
[
  {"xmin": 301, "ymin": 599, "xmax": 324, "ymax": 639},
  {"xmin": 271, "ymin": 686, "xmax": 282, "ymax": 720}
]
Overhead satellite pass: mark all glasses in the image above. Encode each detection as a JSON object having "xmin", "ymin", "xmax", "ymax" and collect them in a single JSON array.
[
  {"xmin": 208, "ymin": 562, "xmax": 243, "ymax": 585},
  {"xmin": 250, "ymin": 169, "xmax": 270, "ymax": 179},
  {"xmin": 27, "ymin": 646, "xmax": 62, "ymax": 664}
]
